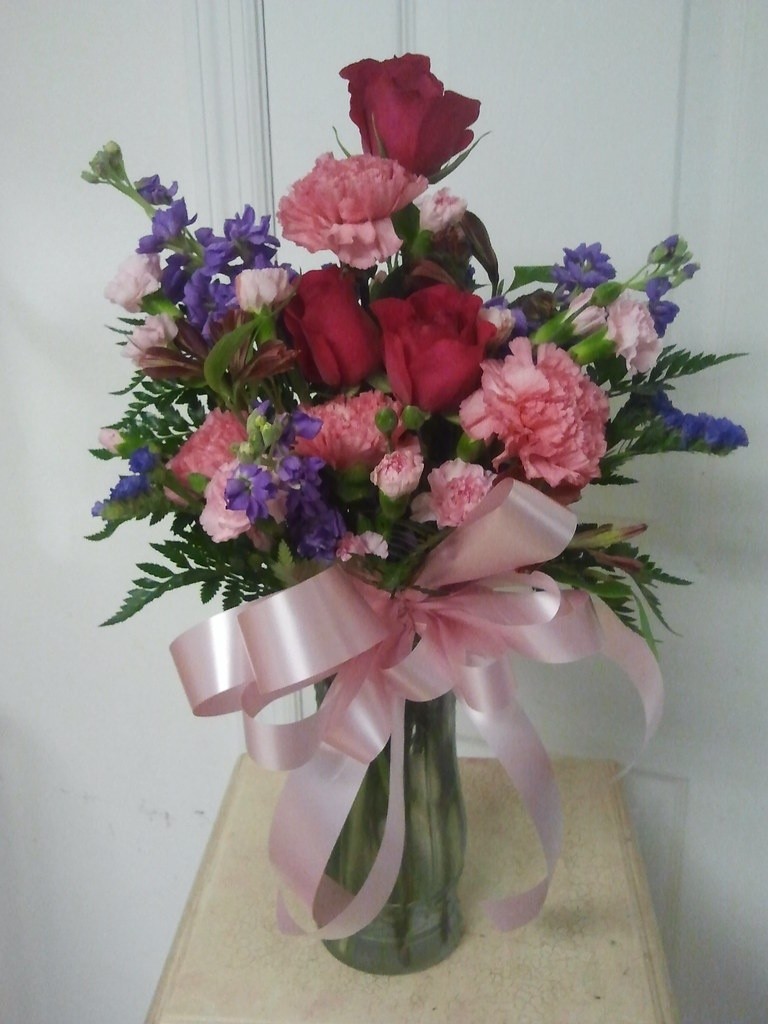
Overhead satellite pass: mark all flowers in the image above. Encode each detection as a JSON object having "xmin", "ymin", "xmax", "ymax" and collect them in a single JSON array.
[{"xmin": 80, "ymin": 49, "xmax": 752, "ymax": 656}]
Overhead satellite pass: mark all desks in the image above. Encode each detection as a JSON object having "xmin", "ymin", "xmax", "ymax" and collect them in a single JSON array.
[{"xmin": 147, "ymin": 756, "xmax": 684, "ymax": 1023}]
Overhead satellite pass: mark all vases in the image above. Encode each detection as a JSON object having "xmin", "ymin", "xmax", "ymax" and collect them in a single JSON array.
[{"xmin": 302, "ymin": 676, "xmax": 466, "ymax": 976}]
